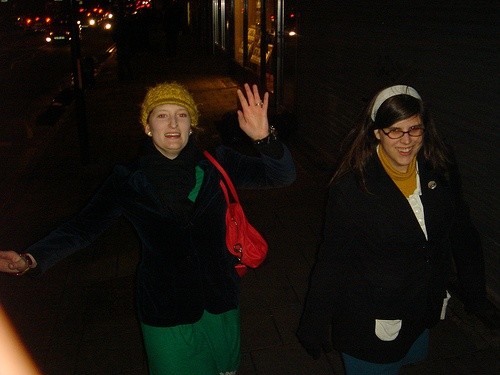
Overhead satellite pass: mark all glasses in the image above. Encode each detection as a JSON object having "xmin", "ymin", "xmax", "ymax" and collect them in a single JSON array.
[{"xmin": 380, "ymin": 127, "xmax": 424, "ymax": 139}]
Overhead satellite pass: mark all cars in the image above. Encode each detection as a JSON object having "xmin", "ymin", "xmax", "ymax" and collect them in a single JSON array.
[{"xmin": 15, "ymin": 6, "xmax": 114, "ymax": 46}]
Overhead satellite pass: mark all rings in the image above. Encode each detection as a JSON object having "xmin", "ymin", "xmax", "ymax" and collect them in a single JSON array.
[{"xmin": 256, "ymin": 103, "xmax": 262, "ymax": 106}]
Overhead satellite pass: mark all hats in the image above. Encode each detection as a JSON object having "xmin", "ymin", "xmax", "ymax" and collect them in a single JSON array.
[{"xmin": 139, "ymin": 81, "xmax": 199, "ymax": 129}]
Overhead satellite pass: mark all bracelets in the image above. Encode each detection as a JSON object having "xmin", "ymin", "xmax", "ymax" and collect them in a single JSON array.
[
  {"xmin": 256, "ymin": 126, "xmax": 278, "ymax": 146},
  {"xmin": 15, "ymin": 254, "xmax": 33, "ymax": 276}
]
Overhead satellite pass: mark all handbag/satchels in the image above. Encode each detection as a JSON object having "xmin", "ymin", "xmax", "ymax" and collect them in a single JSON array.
[{"xmin": 200, "ymin": 147, "xmax": 268, "ymax": 268}]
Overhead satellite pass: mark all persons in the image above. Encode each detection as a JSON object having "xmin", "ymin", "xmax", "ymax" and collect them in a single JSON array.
[
  {"xmin": 0, "ymin": 83, "xmax": 295, "ymax": 375},
  {"xmin": 297, "ymin": 81, "xmax": 486, "ymax": 375}
]
